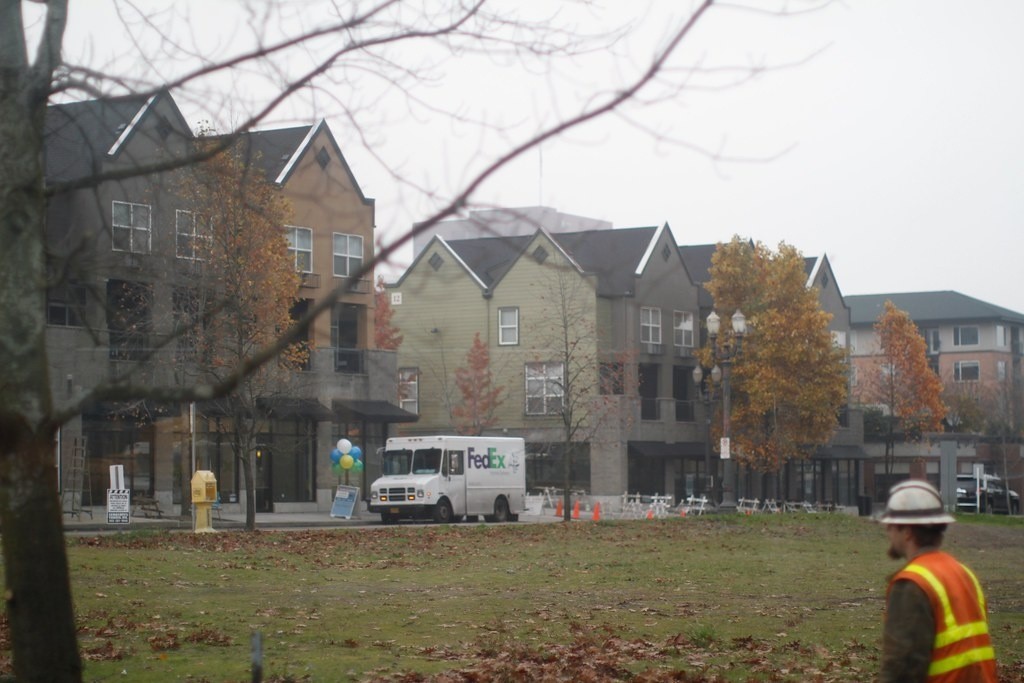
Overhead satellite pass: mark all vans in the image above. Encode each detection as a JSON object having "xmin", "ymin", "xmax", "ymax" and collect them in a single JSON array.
[{"xmin": 367, "ymin": 435, "xmax": 531, "ymax": 524}]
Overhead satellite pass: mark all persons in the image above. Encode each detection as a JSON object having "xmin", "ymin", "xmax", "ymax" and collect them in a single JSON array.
[{"xmin": 878, "ymin": 479, "xmax": 1000, "ymax": 683}]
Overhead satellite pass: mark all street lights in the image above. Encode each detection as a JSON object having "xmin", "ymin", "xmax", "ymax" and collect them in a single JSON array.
[
  {"xmin": 693, "ymin": 358, "xmax": 722, "ymax": 509},
  {"xmin": 706, "ymin": 307, "xmax": 749, "ymax": 515}
]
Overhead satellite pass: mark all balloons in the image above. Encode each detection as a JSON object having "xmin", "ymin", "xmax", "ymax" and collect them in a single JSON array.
[{"xmin": 330, "ymin": 439, "xmax": 364, "ymax": 475}]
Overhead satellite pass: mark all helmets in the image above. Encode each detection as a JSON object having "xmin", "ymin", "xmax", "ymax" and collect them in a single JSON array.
[{"xmin": 867, "ymin": 479, "xmax": 958, "ymax": 524}]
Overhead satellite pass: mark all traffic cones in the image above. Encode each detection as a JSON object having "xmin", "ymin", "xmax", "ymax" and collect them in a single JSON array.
[
  {"xmin": 571, "ymin": 499, "xmax": 580, "ymax": 520},
  {"xmin": 646, "ymin": 508, "xmax": 652, "ymax": 520},
  {"xmin": 554, "ymin": 496, "xmax": 564, "ymax": 517},
  {"xmin": 591, "ymin": 500, "xmax": 600, "ymax": 520}
]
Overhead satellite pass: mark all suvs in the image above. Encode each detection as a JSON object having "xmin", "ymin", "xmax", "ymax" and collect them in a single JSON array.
[{"xmin": 955, "ymin": 473, "xmax": 1021, "ymax": 516}]
